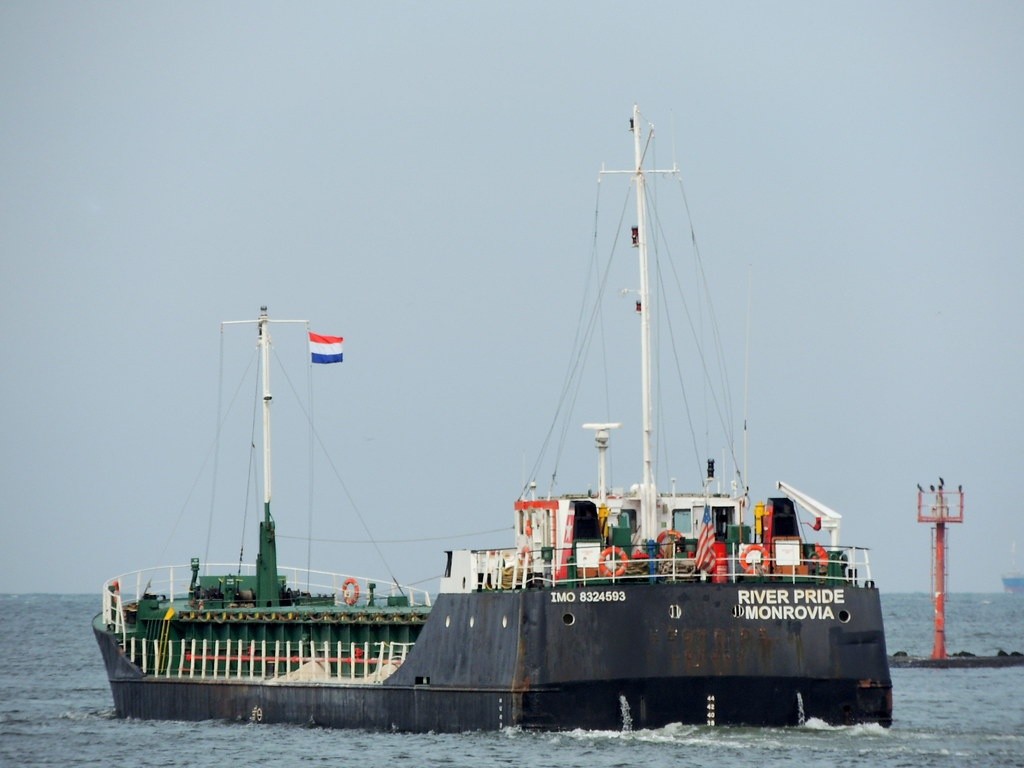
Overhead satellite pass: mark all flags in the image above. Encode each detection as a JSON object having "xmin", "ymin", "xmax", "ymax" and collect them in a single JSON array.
[
  {"xmin": 696, "ymin": 504, "xmax": 716, "ymax": 574},
  {"xmin": 308, "ymin": 332, "xmax": 343, "ymax": 364}
]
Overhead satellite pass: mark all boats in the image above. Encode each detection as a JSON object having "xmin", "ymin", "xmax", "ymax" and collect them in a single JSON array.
[
  {"xmin": 92, "ymin": 101, "xmax": 893, "ymax": 733},
  {"xmin": 1001, "ymin": 578, "xmax": 1023, "ymax": 592}
]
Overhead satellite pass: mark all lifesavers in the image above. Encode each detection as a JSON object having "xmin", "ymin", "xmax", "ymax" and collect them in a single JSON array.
[
  {"xmin": 600, "ymin": 547, "xmax": 628, "ymax": 576},
  {"xmin": 341, "ymin": 579, "xmax": 359, "ymax": 605},
  {"xmin": 813, "ymin": 544, "xmax": 829, "ymax": 569},
  {"xmin": 518, "ymin": 547, "xmax": 531, "ymax": 565},
  {"xmin": 657, "ymin": 531, "xmax": 682, "ymax": 555},
  {"xmin": 112, "ymin": 582, "xmax": 119, "ymax": 603},
  {"xmin": 741, "ymin": 546, "xmax": 771, "ymax": 573}
]
